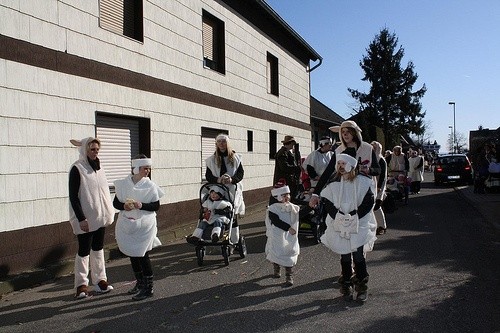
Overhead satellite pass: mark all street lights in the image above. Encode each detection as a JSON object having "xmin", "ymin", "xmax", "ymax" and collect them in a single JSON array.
[
  {"xmin": 448, "ymin": 126, "xmax": 452, "ymax": 151},
  {"xmin": 449, "ymin": 102, "xmax": 456, "ymax": 144}
]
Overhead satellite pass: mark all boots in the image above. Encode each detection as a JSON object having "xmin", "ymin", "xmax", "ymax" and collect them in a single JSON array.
[
  {"xmin": 273, "ymin": 262, "xmax": 281, "ymax": 278},
  {"xmin": 127, "ymin": 273, "xmax": 144, "ymax": 295},
  {"xmin": 132, "ymin": 275, "xmax": 154, "ymax": 300},
  {"xmin": 337, "ymin": 274, "xmax": 353, "ymax": 308},
  {"xmin": 350, "ymin": 273, "xmax": 370, "ymax": 306},
  {"xmin": 284, "ymin": 267, "xmax": 293, "ymax": 286}
]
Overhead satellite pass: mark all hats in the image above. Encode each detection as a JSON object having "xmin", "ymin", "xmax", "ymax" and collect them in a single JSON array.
[
  {"xmin": 271, "ymin": 180, "xmax": 290, "ymax": 202},
  {"xmin": 209, "ymin": 185, "xmax": 226, "ymax": 196},
  {"xmin": 319, "ymin": 136, "xmax": 332, "ymax": 147},
  {"xmin": 335, "ymin": 147, "xmax": 357, "ymax": 172},
  {"xmin": 87, "ymin": 137, "xmax": 100, "ymax": 144},
  {"xmin": 216, "ymin": 134, "xmax": 229, "ymax": 142},
  {"xmin": 281, "ymin": 136, "xmax": 296, "ymax": 145}
]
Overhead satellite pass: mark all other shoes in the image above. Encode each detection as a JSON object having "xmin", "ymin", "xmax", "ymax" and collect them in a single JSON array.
[
  {"xmin": 186, "ymin": 235, "xmax": 202, "ymax": 247},
  {"xmin": 211, "ymin": 233, "xmax": 219, "ymax": 243},
  {"xmin": 377, "ymin": 227, "xmax": 385, "ymax": 235}
]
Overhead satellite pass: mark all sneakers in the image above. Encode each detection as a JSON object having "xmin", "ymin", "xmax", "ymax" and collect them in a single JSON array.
[
  {"xmin": 76, "ymin": 285, "xmax": 88, "ymax": 299},
  {"xmin": 96, "ymin": 280, "xmax": 113, "ymax": 293}
]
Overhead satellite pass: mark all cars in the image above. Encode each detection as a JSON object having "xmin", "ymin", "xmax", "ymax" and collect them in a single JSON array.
[{"xmin": 433, "ymin": 154, "xmax": 473, "ymax": 184}]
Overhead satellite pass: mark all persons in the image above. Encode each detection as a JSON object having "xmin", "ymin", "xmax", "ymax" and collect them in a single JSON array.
[
  {"xmin": 205, "ymin": 133, "xmax": 247, "ymax": 264},
  {"xmin": 113, "ymin": 154, "xmax": 165, "ymax": 300},
  {"xmin": 424, "ymin": 149, "xmax": 437, "ymax": 171},
  {"xmin": 370, "ymin": 141, "xmax": 388, "ymax": 235},
  {"xmin": 293, "ymin": 136, "xmax": 415, "ymax": 243},
  {"xmin": 309, "ymin": 120, "xmax": 381, "ymax": 283},
  {"xmin": 408, "ymin": 151, "xmax": 423, "ymax": 194},
  {"xmin": 69, "ymin": 137, "xmax": 115, "ymax": 298},
  {"xmin": 320, "ymin": 153, "xmax": 376, "ymax": 302},
  {"xmin": 265, "ymin": 179, "xmax": 300, "ymax": 287},
  {"xmin": 472, "ymin": 141, "xmax": 500, "ymax": 194},
  {"xmin": 268, "ymin": 135, "xmax": 304, "ymax": 206},
  {"xmin": 384, "ymin": 145, "xmax": 409, "ymax": 178},
  {"xmin": 186, "ymin": 185, "xmax": 233, "ymax": 245}
]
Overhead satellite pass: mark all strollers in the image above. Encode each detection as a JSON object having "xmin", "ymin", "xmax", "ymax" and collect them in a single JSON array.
[
  {"xmin": 291, "ymin": 189, "xmax": 321, "ymax": 244},
  {"xmin": 194, "ymin": 180, "xmax": 247, "ymax": 265},
  {"xmin": 386, "ymin": 170, "xmax": 407, "ymax": 206}
]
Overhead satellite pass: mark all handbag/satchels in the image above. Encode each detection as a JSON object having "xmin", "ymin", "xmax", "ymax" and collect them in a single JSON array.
[{"xmin": 333, "ymin": 212, "xmax": 359, "ymax": 239}]
